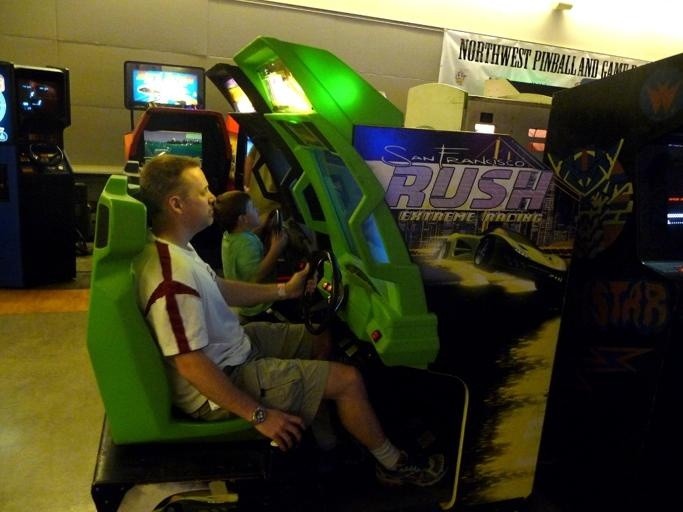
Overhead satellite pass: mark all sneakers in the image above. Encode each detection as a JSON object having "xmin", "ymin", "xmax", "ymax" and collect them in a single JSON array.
[{"xmin": 375, "ymin": 450, "xmax": 448, "ymax": 485}]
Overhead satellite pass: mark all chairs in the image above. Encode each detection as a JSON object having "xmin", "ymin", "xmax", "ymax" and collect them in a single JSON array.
[{"xmin": 86, "ymin": 175, "xmax": 260, "ymax": 446}]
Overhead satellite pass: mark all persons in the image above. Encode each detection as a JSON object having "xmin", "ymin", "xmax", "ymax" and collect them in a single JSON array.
[
  {"xmin": 212, "ymin": 189, "xmax": 295, "ymax": 323},
  {"xmin": 131, "ymin": 153, "xmax": 447, "ymax": 487}
]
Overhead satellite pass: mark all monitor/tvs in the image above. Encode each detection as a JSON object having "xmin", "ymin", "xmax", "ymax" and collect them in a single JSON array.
[
  {"xmin": 662, "ymin": 142, "xmax": 683, "ymax": 226},
  {"xmin": 244, "ymin": 128, "xmax": 300, "ymax": 187},
  {"xmin": 123, "ymin": 60, "xmax": 206, "ymax": 111},
  {"xmin": 142, "ymin": 127, "xmax": 204, "ymax": 170},
  {"xmin": 309, "ymin": 149, "xmax": 389, "ymax": 263},
  {"xmin": 15, "ymin": 78, "xmax": 60, "ymax": 112}
]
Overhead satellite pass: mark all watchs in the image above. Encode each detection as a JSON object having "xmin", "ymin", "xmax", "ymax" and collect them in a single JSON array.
[{"xmin": 249, "ymin": 403, "xmax": 267, "ymax": 426}]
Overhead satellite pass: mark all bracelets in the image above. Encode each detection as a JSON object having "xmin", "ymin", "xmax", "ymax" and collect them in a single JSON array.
[{"xmin": 277, "ymin": 283, "xmax": 287, "ymax": 299}]
